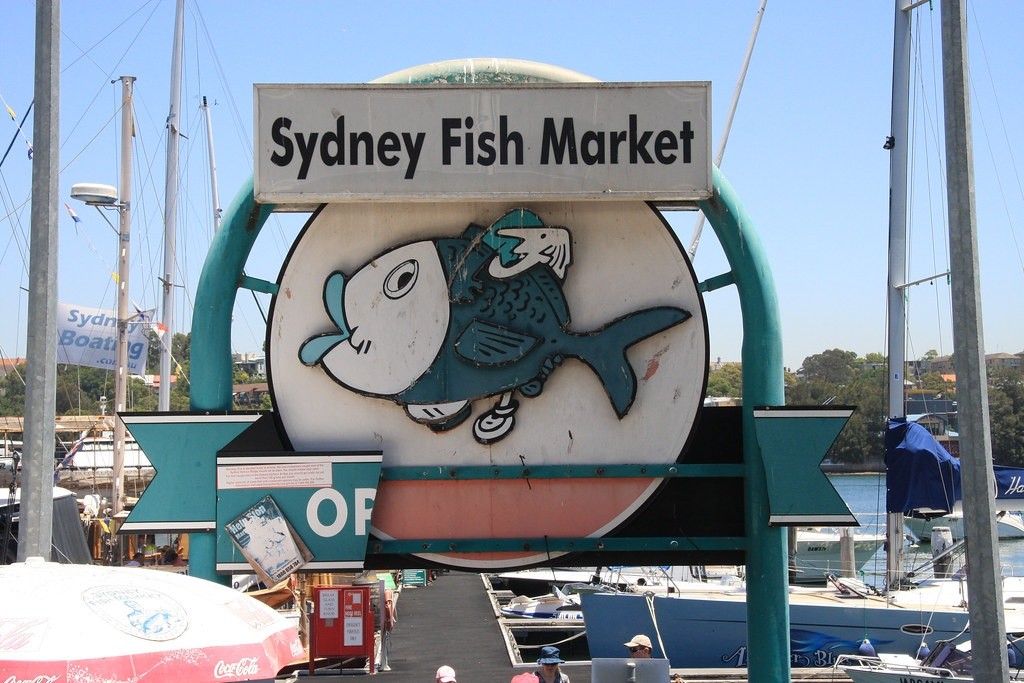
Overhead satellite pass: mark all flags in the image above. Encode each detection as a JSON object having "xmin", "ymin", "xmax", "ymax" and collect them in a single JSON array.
[
  {"xmin": 66, "ymin": 204, "xmax": 83, "ymax": 236},
  {"xmin": 56, "ymin": 301, "xmax": 156, "ymax": 379},
  {"xmin": 152, "ymin": 323, "xmax": 170, "ymax": 339}
]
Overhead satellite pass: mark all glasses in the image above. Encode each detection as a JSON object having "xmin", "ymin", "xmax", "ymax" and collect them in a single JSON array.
[
  {"xmin": 543, "ymin": 663, "xmax": 559, "ymax": 666},
  {"xmin": 628, "ymin": 647, "xmax": 643, "ymax": 652}
]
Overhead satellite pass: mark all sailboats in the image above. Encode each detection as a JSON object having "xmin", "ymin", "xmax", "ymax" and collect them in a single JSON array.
[{"xmin": 0, "ymin": 0, "xmax": 332, "ymax": 610}]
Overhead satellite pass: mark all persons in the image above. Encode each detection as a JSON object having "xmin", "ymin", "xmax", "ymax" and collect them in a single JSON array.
[
  {"xmin": 624, "ymin": 635, "xmax": 653, "ymax": 658},
  {"xmin": 125, "ymin": 553, "xmax": 144, "ymax": 567},
  {"xmin": 434, "ymin": 665, "xmax": 457, "ymax": 683},
  {"xmin": 533, "ymin": 647, "xmax": 571, "ymax": 683},
  {"xmin": 511, "ymin": 672, "xmax": 539, "ymax": 683},
  {"xmin": 157, "ymin": 545, "xmax": 185, "ymax": 565}
]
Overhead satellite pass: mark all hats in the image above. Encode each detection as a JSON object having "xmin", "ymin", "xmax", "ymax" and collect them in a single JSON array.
[
  {"xmin": 623, "ymin": 635, "xmax": 653, "ymax": 649},
  {"xmin": 157, "ymin": 544, "xmax": 173, "ymax": 552},
  {"xmin": 436, "ymin": 665, "xmax": 456, "ymax": 683},
  {"xmin": 164, "ymin": 552, "xmax": 178, "ymax": 561},
  {"xmin": 537, "ymin": 646, "xmax": 565, "ymax": 665}
]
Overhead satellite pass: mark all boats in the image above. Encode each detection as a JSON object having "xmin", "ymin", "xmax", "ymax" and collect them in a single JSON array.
[
  {"xmin": 492, "ymin": 570, "xmax": 746, "ymax": 631},
  {"xmin": 792, "ymin": 529, "xmax": 887, "ymax": 585},
  {"xmin": 576, "ymin": 588, "xmax": 971, "ymax": 668},
  {"xmin": 916, "ymin": 507, "xmax": 1024, "ymax": 542},
  {"xmin": 840, "ymin": 667, "xmax": 975, "ymax": 683}
]
